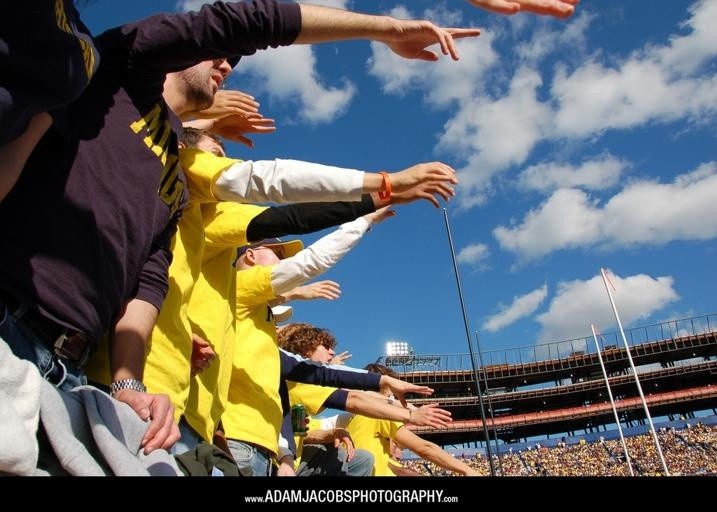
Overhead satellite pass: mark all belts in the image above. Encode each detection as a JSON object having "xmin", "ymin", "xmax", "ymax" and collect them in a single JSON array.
[{"xmin": 5, "ymin": 293, "xmax": 92, "ymax": 371}]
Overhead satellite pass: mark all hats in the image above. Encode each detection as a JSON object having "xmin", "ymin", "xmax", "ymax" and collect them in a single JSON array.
[
  {"xmin": 271, "ymin": 305, "xmax": 293, "ymax": 323},
  {"xmin": 233, "ymin": 237, "xmax": 305, "ymax": 267}
]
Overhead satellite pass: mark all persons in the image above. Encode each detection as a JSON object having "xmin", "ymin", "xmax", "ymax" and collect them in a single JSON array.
[
  {"xmin": 0, "ymin": 0, "xmax": 486, "ymax": 475},
  {"xmin": 483, "ymin": 416, "xmax": 716, "ymax": 477},
  {"xmin": 470, "ymin": 0, "xmax": 577, "ymax": 19}
]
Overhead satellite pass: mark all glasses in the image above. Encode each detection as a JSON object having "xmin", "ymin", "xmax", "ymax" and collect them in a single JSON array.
[{"xmin": 254, "ymin": 247, "xmax": 283, "ymax": 260}]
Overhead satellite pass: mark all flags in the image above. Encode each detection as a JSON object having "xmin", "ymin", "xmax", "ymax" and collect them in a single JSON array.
[
  {"xmin": 590, "ymin": 324, "xmax": 606, "ymax": 342},
  {"xmin": 601, "ymin": 269, "xmax": 618, "ymax": 292}
]
